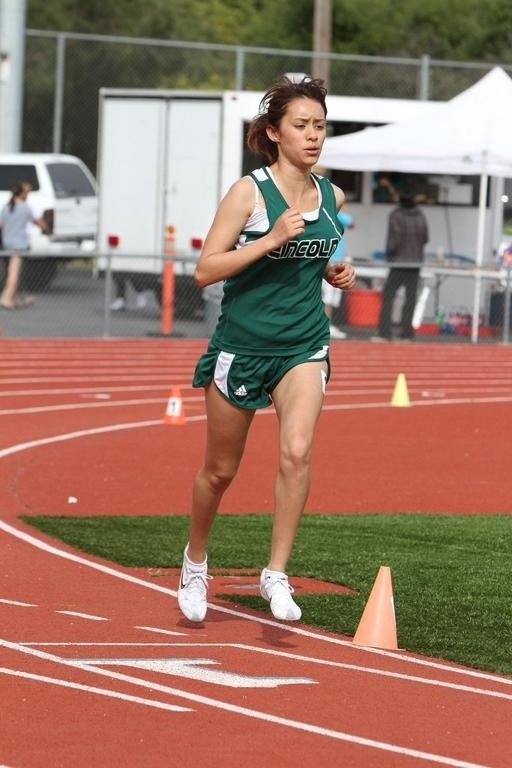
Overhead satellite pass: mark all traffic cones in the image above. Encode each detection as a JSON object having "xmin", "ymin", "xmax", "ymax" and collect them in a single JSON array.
[
  {"xmin": 350, "ymin": 563, "xmax": 408, "ymax": 652},
  {"xmin": 390, "ymin": 372, "xmax": 412, "ymax": 408},
  {"xmin": 164, "ymin": 385, "xmax": 186, "ymax": 425}
]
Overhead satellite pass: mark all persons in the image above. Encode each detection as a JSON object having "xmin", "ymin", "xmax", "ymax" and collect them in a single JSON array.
[
  {"xmin": 319, "ymin": 204, "xmax": 356, "ymax": 341},
  {"xmin": 0, "ymin": 179, "xmax": 52, "ymax": 311},
  {"xmin": 370, "ymin": 186, "xmax": 432, "ymax": 344},
  {"xmin": 178, "ymin": 74, "xmax": 359, "ymax": 625}
]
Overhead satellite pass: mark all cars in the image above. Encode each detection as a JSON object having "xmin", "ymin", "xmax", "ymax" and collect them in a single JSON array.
[{"xmin": 1, "ymin": 152, "xmax": 98, "ymax": 294}]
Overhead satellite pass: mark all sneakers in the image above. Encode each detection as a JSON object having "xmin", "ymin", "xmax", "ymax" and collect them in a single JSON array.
[
  {"xmin": 176, "ymin": 541, "xmax": 209, "ymax": 623},
  {"xmin": 328, "ymin": 323, "xmax": 347, "ymax": 340},
  {"xmin": 259, "ymin": 567, "xmax": 302, "ymax": 623}
]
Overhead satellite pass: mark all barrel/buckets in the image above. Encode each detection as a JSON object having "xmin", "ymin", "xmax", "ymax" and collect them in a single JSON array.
[{"xmin": 343, "ymin": 289, "xmax": 383, "ymax": 328}]
